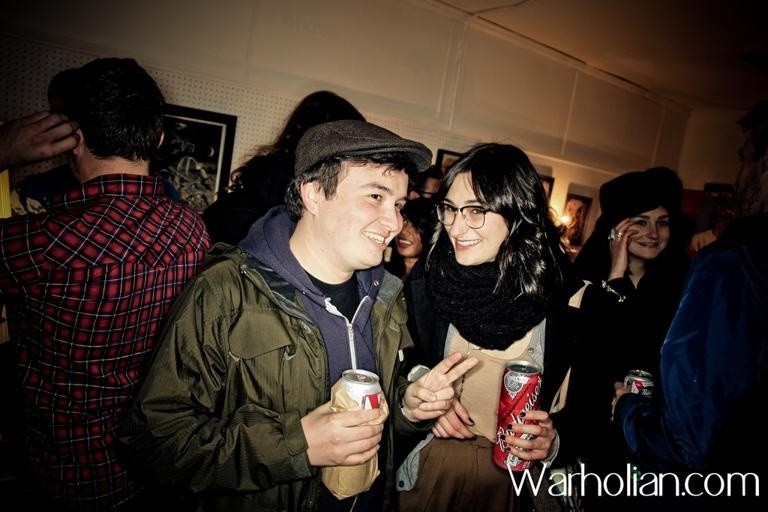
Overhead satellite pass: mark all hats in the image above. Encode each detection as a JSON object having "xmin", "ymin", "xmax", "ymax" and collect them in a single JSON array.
[
  {"xmin": 593, "ymin": 169, "xmax": 675, "ymax": 234},
  {"xmin": 293, "ymin": 118, "xmax": 434, "ymax": 179}
]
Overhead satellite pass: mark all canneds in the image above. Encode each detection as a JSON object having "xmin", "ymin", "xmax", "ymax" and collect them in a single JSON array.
[
  {"xmin": 340, "ymin": 370, "xmax": 382, "ymax": 411},
  {"xmin": 624, "ymin": 369, "xmax": 655, "ymax": 399},
  {"xmin": 493, "ymin": 359, "xmax": 542, "ymax": 471}
]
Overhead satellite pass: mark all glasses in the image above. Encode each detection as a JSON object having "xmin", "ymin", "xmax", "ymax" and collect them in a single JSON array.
[{"xmin": 435, "ymin": 201, "xmax": 492, "ymax": 230}]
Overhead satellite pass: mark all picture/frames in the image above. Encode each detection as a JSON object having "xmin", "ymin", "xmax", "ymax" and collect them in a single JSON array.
[
  {"xmin": 564, "ymin": 193, "xmax": 592, "ymax": 250},
  {"xmin": 435, "ymin": 148, "xmax": 554, "ymax": 207},
  {"xmin": 152, "ymin": 104, "xmax": 237, "ymax": 213}
]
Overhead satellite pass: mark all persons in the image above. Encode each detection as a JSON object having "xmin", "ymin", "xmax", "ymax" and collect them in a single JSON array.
[
  {"xmin": 114, "ymin": 121, "xmax": 430, "ymax": 512},
  {"xmin": 198, "ymin": 89, "xmax": 765, "ymax": 511},
  {"xmin": 0, "ymin": 56, "xmax": 212, "ymax": 512}
]
